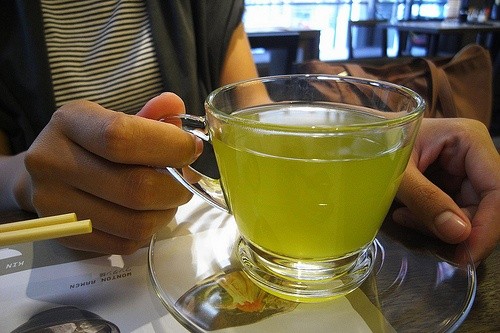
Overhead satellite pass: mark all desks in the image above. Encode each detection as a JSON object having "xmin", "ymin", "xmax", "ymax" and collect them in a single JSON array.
[{"xmin": 246, "ymin": 19, "xmax": 500, "ymax": 63}]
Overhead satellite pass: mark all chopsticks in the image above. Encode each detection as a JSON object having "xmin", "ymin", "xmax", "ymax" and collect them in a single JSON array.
[{"xmin": 0, "ymin": 213, "xmax": 93, "ymax": 246}]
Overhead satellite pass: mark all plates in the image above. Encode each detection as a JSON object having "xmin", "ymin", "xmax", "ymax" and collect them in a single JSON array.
[{"xmin": 148, "ymin": 207, "xmax": 477, "ymax": 333}]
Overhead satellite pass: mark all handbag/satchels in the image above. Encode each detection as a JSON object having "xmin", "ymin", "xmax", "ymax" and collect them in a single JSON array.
[{"xmin": 290, "ymin": 43, "xmax": 493, "ymax": 130}]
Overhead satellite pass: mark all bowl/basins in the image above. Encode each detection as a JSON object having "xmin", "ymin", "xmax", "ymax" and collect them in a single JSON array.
[{"xmin": 10, "ymin": 306, "xmax": 121, "ymax": 333}]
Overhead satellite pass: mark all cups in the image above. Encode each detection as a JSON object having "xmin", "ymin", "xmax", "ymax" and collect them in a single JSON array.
[{"xmin": 158, "ymin": 74, "xmax": 427, "ymax": 303}]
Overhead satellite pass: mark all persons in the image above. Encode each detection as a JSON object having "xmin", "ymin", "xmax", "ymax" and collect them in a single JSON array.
[{"xmin": 0, "ymin": 0, "xmax": 500, "ymax": 275}]
